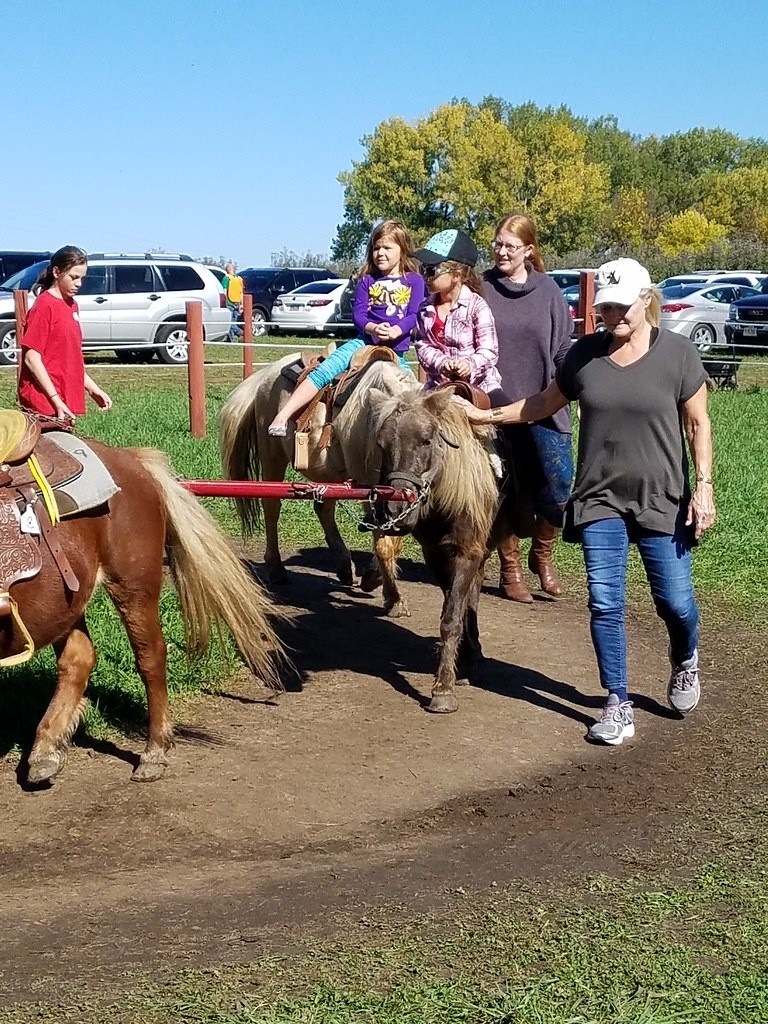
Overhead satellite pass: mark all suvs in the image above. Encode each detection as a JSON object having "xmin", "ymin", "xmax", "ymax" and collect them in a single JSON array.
[
  {"xmin": 236, "ymin": 267, "xmax": 339, "ymax": 338},
  {"xmin": 0, "ymin": 253, "xmax": 232, "ymax": 365}
]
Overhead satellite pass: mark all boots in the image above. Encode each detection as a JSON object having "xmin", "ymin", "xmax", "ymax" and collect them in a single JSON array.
[
  {"xmin": 495, "ymin": 515, "xmax": 534, "ymax": 604},
  {"xmin": 528, "ymin": 503, "xmax": 564, "ymax": 596}
]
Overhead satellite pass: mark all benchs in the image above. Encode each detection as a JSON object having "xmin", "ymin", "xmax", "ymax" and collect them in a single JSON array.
[{"xmin": 701, "ymin": 360, "xmax": 768, "ymax": 388}]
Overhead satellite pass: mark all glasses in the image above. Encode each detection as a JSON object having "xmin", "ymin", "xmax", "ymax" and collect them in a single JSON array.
[
  {"xmin": 416, "ymin": 263, "xmax": 458, "ymax": 280},
  {"xmin": 489, "ymin": 239, "xmax": 530, "ymax": 254}
]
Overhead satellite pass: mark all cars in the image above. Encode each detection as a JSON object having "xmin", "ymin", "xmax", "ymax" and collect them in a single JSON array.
[
  {"xmin": 544, "ymin": 267, "xmax": 608, "ymax": 334},
  {"xmin": 658, "ymin": 283, "xmax": 763, "ymax": 352},
  {"xmin": 657, "ymin": 269, "xmax": 768, "ymax": 293},
  {"xmin": 269, "ymin": 278, "xmax": 359, "ymax": 338},
  {"xmin": 723, "ymin": 294, "xmax": 768, "ymax": 353}
]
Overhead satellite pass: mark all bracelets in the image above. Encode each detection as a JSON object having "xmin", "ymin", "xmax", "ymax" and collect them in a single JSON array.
[
  {"xmin": 490, "ymin": 408, "xmax": 493, "ymax": 419},
  {"xmin": 49, "ymin": 394, "xmax": 58, "ymax": 400},
  {"xmin": 697, "ymin": 478, "xmax": 713, "ymax": 484}
]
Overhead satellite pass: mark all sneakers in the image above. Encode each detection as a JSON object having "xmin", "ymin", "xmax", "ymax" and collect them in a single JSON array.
[
  {"xmin": 666, "ymin": 641, "xmax": 700, "ymax": 714},
  {"xmin": 587, "ymin": 692, "xmax": 636, "ymax": 745}
]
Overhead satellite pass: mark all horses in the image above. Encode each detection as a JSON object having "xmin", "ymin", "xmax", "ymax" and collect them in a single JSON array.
[
  {"xmin": 0, "ymin": 409, "xmax": 303, "ymax": 781},
  {"xmin": 217, "ymin": 338, "xmax": 425, "ymax": 592},
  {"xmin": 367, "ymin": 385, "xmax": 503, "ymax": 712}
]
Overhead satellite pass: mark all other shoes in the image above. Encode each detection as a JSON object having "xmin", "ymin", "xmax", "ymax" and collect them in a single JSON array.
[{"xmin": 269, "ymin": 422, "xmax": 289, "ymax": 437}]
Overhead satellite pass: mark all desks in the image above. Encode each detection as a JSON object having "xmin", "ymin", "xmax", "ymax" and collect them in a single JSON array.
[{"xmin": 691, "ymin": 341, "xmax": 768, "ymax": 386}]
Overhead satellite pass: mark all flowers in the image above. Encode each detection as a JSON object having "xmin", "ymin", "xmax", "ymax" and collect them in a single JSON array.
[
  {"xmin": 369, "ymin": 284, "xmax": 383, "ymax": 297},
  {"xmin": 393, "ymin": 287, "xmax": 412, "ymax": 305}
]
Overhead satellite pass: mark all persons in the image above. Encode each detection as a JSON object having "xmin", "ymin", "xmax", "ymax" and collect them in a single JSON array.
[
  {"xmin": 268, "ymin": 219, "xmax": 424, "ymax": 437},
  {"xmin": 480, "ymin": 214, "xmax": 574, "ymax": 604},
  {"xmin": 18, "ymin": 246, "xmax": 112, "ymax": 435},
  {"xmin": 448, "ymin": 257, "xmax": 717, "ymax": 747},
  {"xmin": 358, "ymin": 229, "xmax": 563, "ymax": 531},
  {"xmin": 222, "ymin": 264, "xmax": 243, "ymax": 343}
]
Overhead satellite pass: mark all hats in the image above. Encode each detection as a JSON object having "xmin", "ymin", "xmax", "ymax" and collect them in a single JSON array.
[
  {"xmin": 590, "ymin": 258, "xmax": 653, "ymax": 306},
  {"xmin": 407, "ymin": 227, "xmax": 479, "ymax": 269}
]
